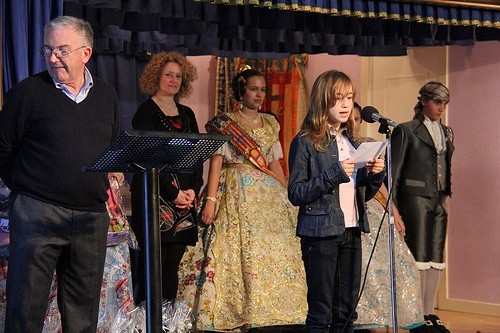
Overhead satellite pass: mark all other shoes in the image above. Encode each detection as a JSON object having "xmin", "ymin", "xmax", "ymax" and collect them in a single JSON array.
[{"xmin": 411, "ymin": 313, "xmax": 450, "ymax": 333}]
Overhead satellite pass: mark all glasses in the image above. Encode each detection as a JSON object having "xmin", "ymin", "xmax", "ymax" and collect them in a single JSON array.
[{"xmin": 40, "ymin": 46, "xmax": 86, "ymax": 58}]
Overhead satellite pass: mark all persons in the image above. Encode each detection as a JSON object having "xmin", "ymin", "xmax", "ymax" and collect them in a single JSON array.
[
  {"xmin": 349, "ymin": 100, "xmax": 426, "ymax": 333},
  {"xmin": 176, "ymin": 69, "xmax": 309, "ymax": 333},
  {"xmin": 0, "ymin": 15, "xmax": 121, "ymax": 333},
  {"xmin": 130, "ymin": 51, "xmax": 204, "ymax": 310},
  {"xmin": 287, "ymin": 69, "xmax": 386, "ymax": 333},
  {"xmin": 385, "ymin": 81, "xmax": 455, "ymax": 333}
]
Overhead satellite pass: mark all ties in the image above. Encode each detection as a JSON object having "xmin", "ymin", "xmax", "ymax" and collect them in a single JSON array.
[{"xmin": 431, "ymin": 124, "xmax": 442, "ymax": 154}]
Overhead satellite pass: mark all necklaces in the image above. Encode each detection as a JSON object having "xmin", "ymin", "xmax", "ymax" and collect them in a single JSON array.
[{"xmin": 238, "ymin": 110, "xmax": 261, "ymax": 124}]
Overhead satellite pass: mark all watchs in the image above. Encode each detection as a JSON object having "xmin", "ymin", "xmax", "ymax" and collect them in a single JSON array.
[{"xmin": 206, "ymin": 196, "xmax": 218, "ymax": 202}]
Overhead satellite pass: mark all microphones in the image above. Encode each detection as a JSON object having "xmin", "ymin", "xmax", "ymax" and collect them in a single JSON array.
[{"xmin": 361, "ymin": 106, "xmax": 401, "ymax": 129}]
